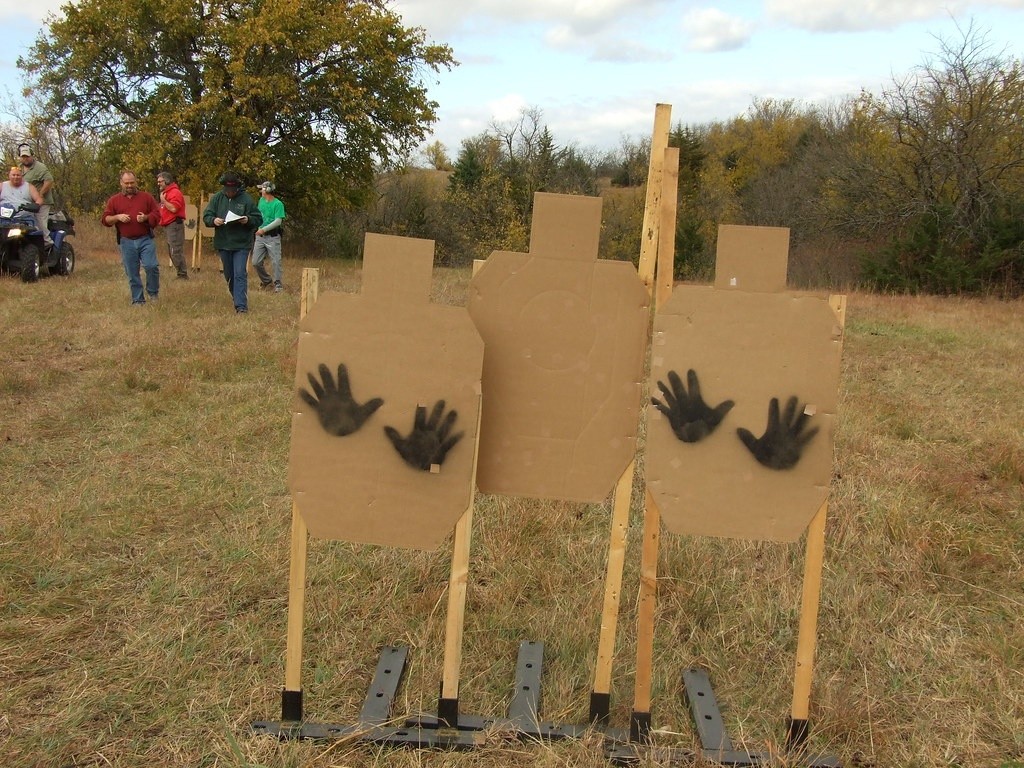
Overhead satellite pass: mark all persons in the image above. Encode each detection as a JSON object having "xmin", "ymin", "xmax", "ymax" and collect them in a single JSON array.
[
  {"xmin": 17, "ymin": 143, "xmax": 55, "ymax": 252},
  {"xmin": 157, "ymin": 172, "xmax": 189, "ymax": 282},
  {"xmin": 251, "ymin": 181, "xmax": 286, "ymax": 294},
  {"xmin": 0, "ymin": 166, "xmax": 44, "ymax": 231},
  {"xmin": 203, "ymin": 170, "xmax": 263, "ymax": 314},
  {"xmin": 102, "ymin": 172, "xmax": 161, "ymax": 308}
]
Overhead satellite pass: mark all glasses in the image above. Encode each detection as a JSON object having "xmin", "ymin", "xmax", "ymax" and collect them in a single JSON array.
[
  {"xmin": 11, "ymin": 167, "xmax": 22, "ymax": 171},
  {"xmin": 157, "ymin": 180, "xmax": 165, "ymax": 183},
  {"xmin": 120, "ymin": 178, "xmax": 135, "ymax": 185}
]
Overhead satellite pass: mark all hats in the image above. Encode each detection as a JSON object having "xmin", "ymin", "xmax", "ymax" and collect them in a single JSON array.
[
  {"xmin": 257, "ymin": 181, "xmax": 276, "ymax": 191},
  {"xmin": 18, "ymin": 143, "xmax": 34, "ymax": 156},
  {"xmin": 219, "ymin": 174, "xmax": 241, "ymax": 188}
]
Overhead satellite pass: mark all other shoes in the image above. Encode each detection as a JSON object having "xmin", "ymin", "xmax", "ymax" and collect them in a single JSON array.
[
  {"xmin": 258, "ymin": 279, "xmax": 273, "ymax": 291},
  {"xmin": 275, "ymin": 284, "xmax": 283, "ymax": 293},
  {"xmin": 149, "ymin": 295, "xmax": 158, "ymax": 305}
]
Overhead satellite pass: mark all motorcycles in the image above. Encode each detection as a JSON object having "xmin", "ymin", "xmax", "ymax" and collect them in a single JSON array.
[{"xmin": 0, "ymin": 203, "xmax": 76, "ymax": 283}]
